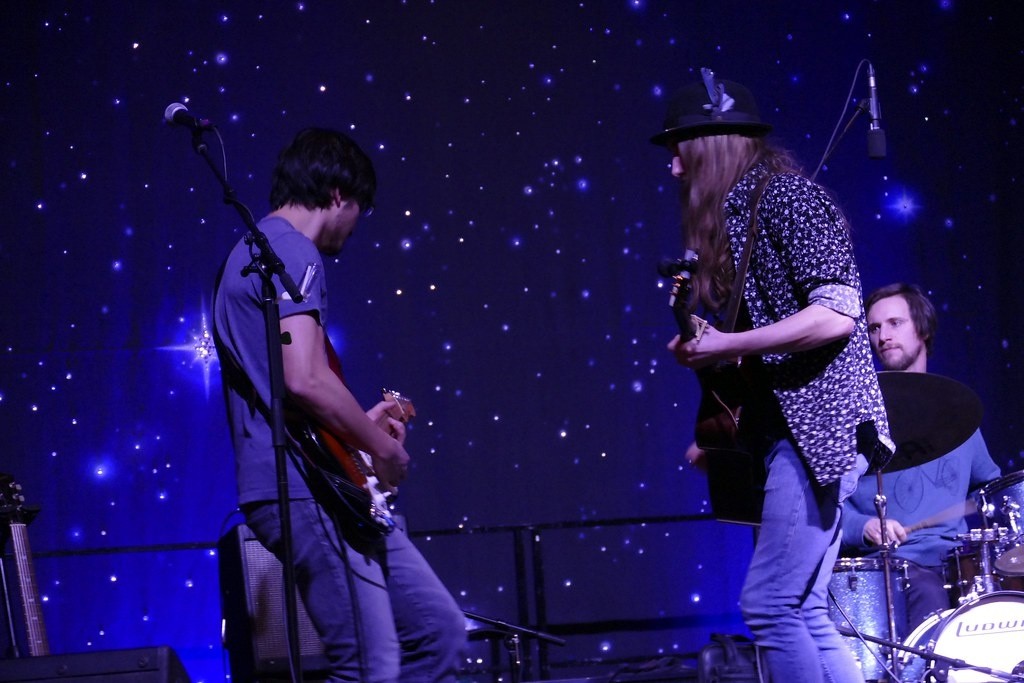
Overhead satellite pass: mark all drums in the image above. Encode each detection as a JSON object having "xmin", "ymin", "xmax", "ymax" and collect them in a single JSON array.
[
  {"xmin": 827, "ymin": 554, "xmax": 914, "ymax": 683},
  {"xmin": 897, "ymin": 586, "xmax": 1024, "ymax": 682},
  {"xmin": 970, "ymin": 470, "xmax": 1024, "ymax": 579}
]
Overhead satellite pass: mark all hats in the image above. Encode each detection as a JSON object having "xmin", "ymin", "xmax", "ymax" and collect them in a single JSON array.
[{"xmin": 648, "ymin": 80, "xmax": 775, "ymax": 147}]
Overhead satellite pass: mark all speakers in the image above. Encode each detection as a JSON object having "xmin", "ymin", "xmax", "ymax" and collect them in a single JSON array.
[
  {"xmin": 217, "ymin": 523, "xmax": 329, "ymax": 683},
  {"xmin": 0, "ymin": 644, "xmax": 191, "ymax": 683}
]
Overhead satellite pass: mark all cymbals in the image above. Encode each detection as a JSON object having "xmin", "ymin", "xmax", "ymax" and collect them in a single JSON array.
[{"xmin": 855, "ymin": 370, "xmax": 980, "ymax": 476}]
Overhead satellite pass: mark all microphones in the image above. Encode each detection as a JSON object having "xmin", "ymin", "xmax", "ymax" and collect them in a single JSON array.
[
  {"xmin": 164, "ymin": 103, "xmax": 217, "ymax": 131},
  {"xmin": 994, "ymin": 665, "xmax": 1024, "ymax": 683},
  {"xmin": 867, "ymin": 61, "xmax": 888, "ymax": 159}
]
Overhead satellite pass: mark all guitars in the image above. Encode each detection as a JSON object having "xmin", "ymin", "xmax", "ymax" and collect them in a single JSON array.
[
  {"xmin": 0, "ymin": 476, "xmax": 53, "ymax": 657},
  {"xmin": 302, "ymin": 385, "xmax": 416, "ymax": 557},
  {"xmin": 655, "ymin": 251, "xmax": 769, "ymax": 520}
]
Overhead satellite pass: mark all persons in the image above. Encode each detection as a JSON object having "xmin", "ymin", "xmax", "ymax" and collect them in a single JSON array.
[
  {"xmin": 209, "ymin": 125, "xmax": 468, "ymax": 683},
  {"xmin": 839, "ymin": 280, "xmax": 1006, "ymax": 626},
  {"xmin": 652, "ymin": 73, "xmax": 896, "ymax": 683}
]
